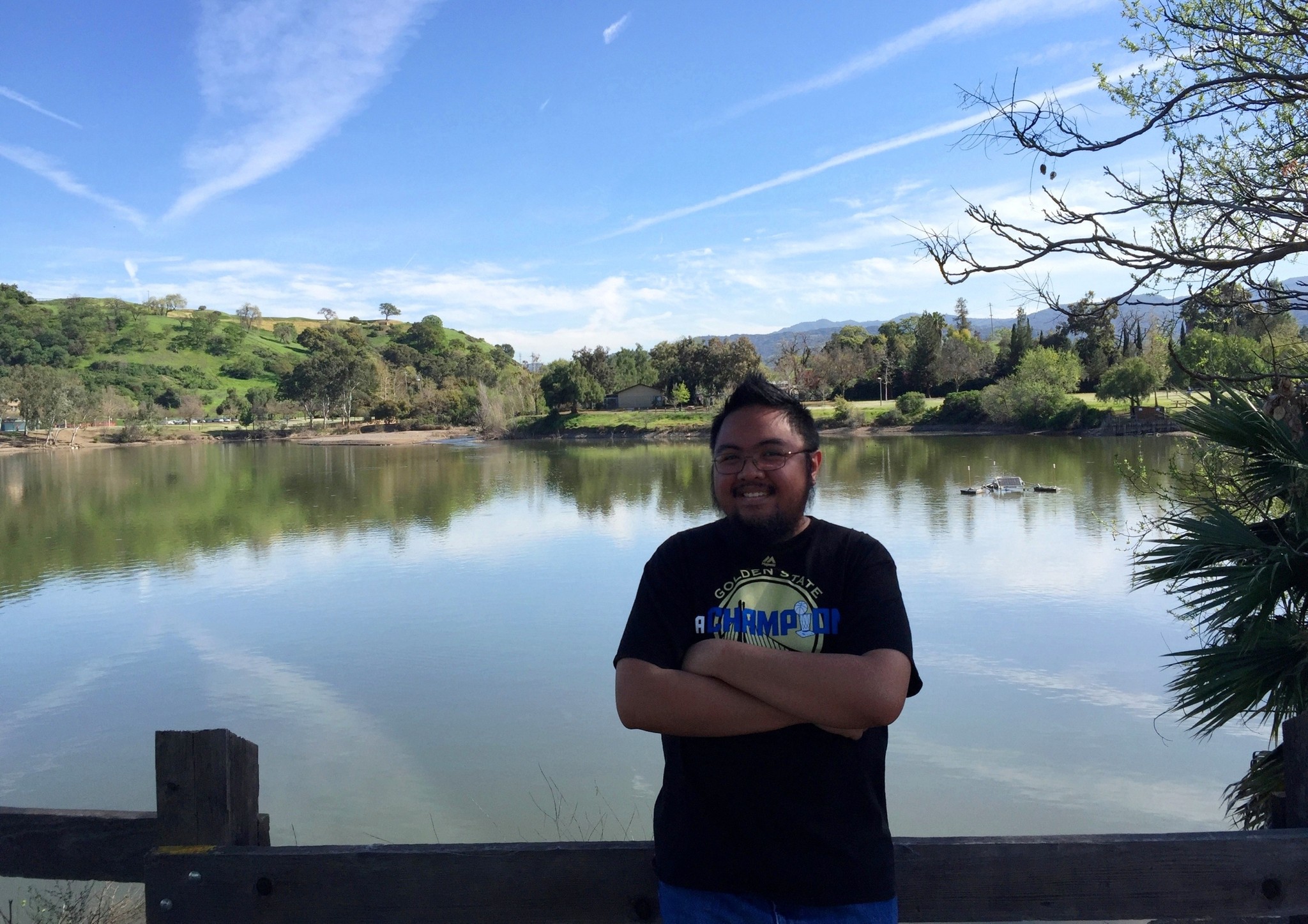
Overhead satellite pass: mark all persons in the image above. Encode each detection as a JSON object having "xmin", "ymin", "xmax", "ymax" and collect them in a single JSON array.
[{"xmin": 612, "ymin": 369, "xmax": 923, "ymax": 923}]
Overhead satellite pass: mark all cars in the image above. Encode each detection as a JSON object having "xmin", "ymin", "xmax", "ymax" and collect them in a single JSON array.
[{"xmin": 158, "ymin": 418, "xmax": 239, "ymax": 425}]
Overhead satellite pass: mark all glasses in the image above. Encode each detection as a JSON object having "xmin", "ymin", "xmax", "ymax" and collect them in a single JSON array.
[{"xmin": 711, "ymin": 446, "xmax": 809, "ymax": 475}]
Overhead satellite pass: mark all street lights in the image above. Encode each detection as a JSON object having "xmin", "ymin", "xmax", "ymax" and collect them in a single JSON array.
[{"xmin": 877, "ymin": 377, "xmax": 882, "ymax": 406}]
